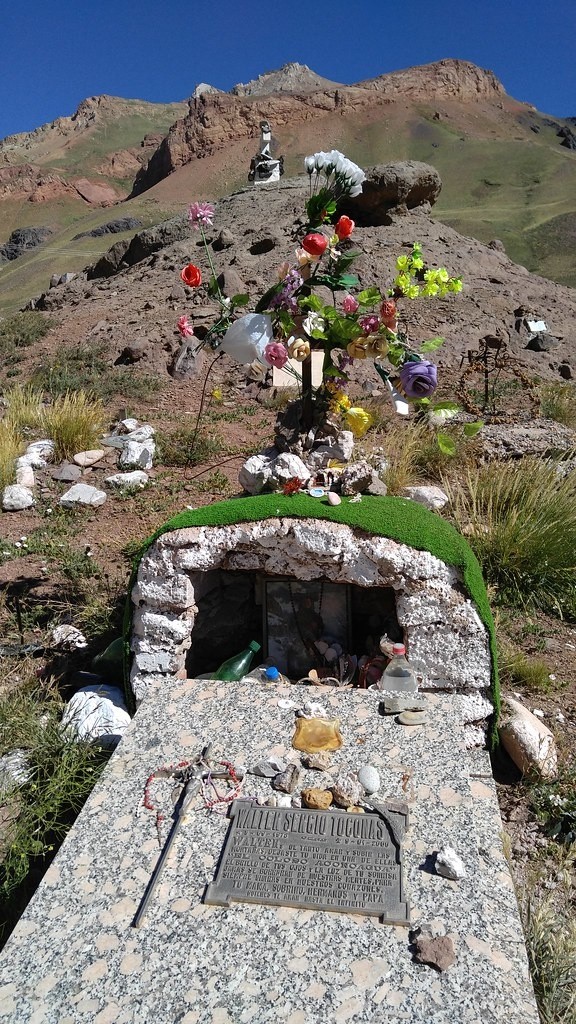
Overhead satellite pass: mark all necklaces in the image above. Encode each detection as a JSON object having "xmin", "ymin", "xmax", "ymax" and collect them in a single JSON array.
[{"xmin": 289, "ymin": 580, "xmax": 324, "ymax": 659}]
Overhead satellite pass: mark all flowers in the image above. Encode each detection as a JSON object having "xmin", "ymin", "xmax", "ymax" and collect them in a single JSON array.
[{"xmin": 176, "ymin": 148, "xmax": 485, "ymax": 457}]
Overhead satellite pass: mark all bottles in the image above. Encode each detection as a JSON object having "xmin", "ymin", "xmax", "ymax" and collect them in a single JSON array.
[
  {"xmin": 210, "ymin": 640, "xmax": 261, "ymax": 682},
  {"xmin": 380, "ymin": 643, "xmax": 419, "ymax": 693},
  {"xmin": 262, "ymin": 667, "xmax": 291, "ymax": 684}
]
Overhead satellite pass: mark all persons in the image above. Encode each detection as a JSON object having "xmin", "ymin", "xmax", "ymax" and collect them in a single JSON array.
[{"xmin": 275, "ymin": 587, "xmax": 325, "ymax": 683}]
